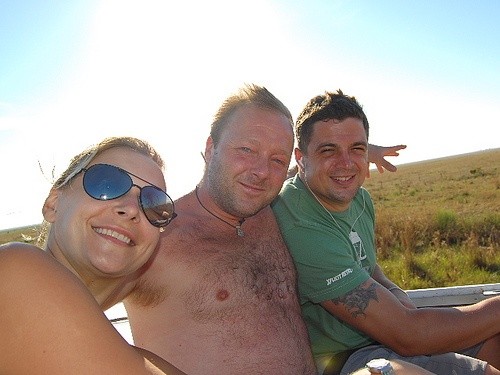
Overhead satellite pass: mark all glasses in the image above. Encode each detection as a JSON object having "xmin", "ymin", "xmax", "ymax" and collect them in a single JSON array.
[{"xmin": 82, "ymin": 164, "xmax": 177, "ymax": 228}]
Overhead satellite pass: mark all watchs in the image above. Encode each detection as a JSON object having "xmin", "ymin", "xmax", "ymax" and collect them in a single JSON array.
[{"xmin": 365, "ymin": 358, "xmax": 394, "ymax": 375}]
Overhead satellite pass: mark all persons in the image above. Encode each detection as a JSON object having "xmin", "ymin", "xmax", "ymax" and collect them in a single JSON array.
[
  {"xmin": 270, "ymin": 88, "xmax": 500, "ymax": 375},
  {"xmin": 99, "ymin": 81, "xmax": 407, "ymax": 375},
  {"xmin": 0, "ymin": 137, "xmax": 436, "ymax": 375}
]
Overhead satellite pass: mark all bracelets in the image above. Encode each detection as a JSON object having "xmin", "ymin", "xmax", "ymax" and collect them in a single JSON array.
[{"xmin": 389, "ymin": 286, "xmax": 411, "ymax": 297}]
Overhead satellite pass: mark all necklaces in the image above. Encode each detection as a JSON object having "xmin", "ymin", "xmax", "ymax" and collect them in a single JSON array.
[
  {"xmin": 301, "ymin": 175, "xmax": 367, "ymax": 261},
  {"xmin": 195, "ymin": 186, "xmax": 246, "ymax": 238}
]
